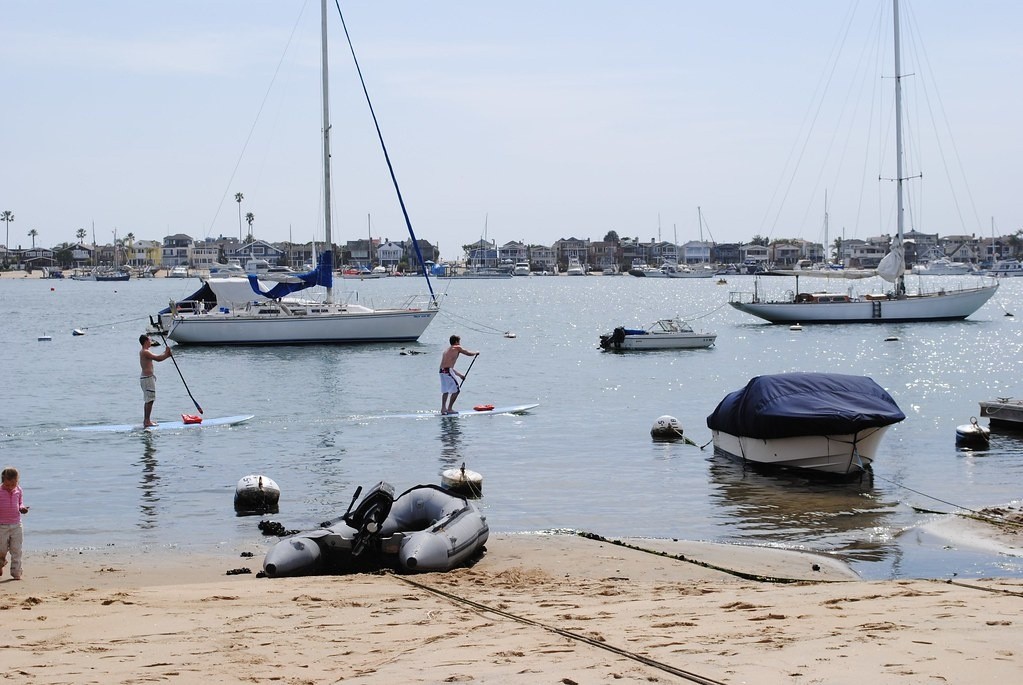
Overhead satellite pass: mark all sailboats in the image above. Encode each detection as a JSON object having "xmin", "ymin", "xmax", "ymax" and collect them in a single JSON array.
[
  {"xmin": 153, "ymin": 0, "xmax": 446, "ymax": 346},
  {"xmin": 725, "ymin": 0, "xmax": 1002, "ymax": 325},
  {"xmin": 629, "ymin": 206, "xmax": 719, "ymax": 278}
]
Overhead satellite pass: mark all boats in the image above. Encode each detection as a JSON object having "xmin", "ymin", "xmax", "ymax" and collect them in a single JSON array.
[
  {"xmin": 972, "ymin": 258, "xmax": 1023, "ymax": 278},
  {"xmin": 514, "ymin": 262, "xmax": 530, "ymax": 276},
  {"xmin": 706, "ymin": 372, "xmax": 906, "ymax": 483},
  {"xmin": 71, "ymin": 262, "xmax": 134, "ymax": 281},
  {"xmin": 599, "ymin": 316, "xmax": 717, "ymax": 353},
  {"xmin": 910, "ymin": 258, "xmax": 971, "ymax": 276},
  {"xmin": 165, "ymin": 253, "xmax": 391, "ymax": 280},
  {"xmin": 568, "ymin": 244, "xmax": 583, "ymax": 276},
  {"xmin": 262, "ymin": 482, "xmax": 487, "ymax": 576}
]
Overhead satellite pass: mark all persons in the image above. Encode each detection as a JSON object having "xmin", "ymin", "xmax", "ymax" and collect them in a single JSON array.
[
  {"xmin": 439, "ymin": 335, "xmax": 479, "ymax": 415},
  {"xmin": 139, "ymin": 334, "xmax": 173, "ymax": 426},
  {"xmin": 0, "ymin": 467, "xmax": 30, "ymax": 580}
]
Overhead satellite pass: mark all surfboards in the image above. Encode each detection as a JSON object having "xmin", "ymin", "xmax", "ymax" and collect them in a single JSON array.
[
  {"xmin": 62, "ymin": 412, "xmax": 257, "ymax": 435},
  {"xmin": 374, "ymin": 400, "xmax": 543, "ymax": 419}
]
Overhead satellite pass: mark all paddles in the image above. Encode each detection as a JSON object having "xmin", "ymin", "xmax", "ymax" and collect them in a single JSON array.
[
  {"xmin": 156, "ymin": 322, "xmax": 206, "ymax": 414},
  {"xmin": 441, "ymin": 350, "xmax": 482, "ymax": 410}
]
